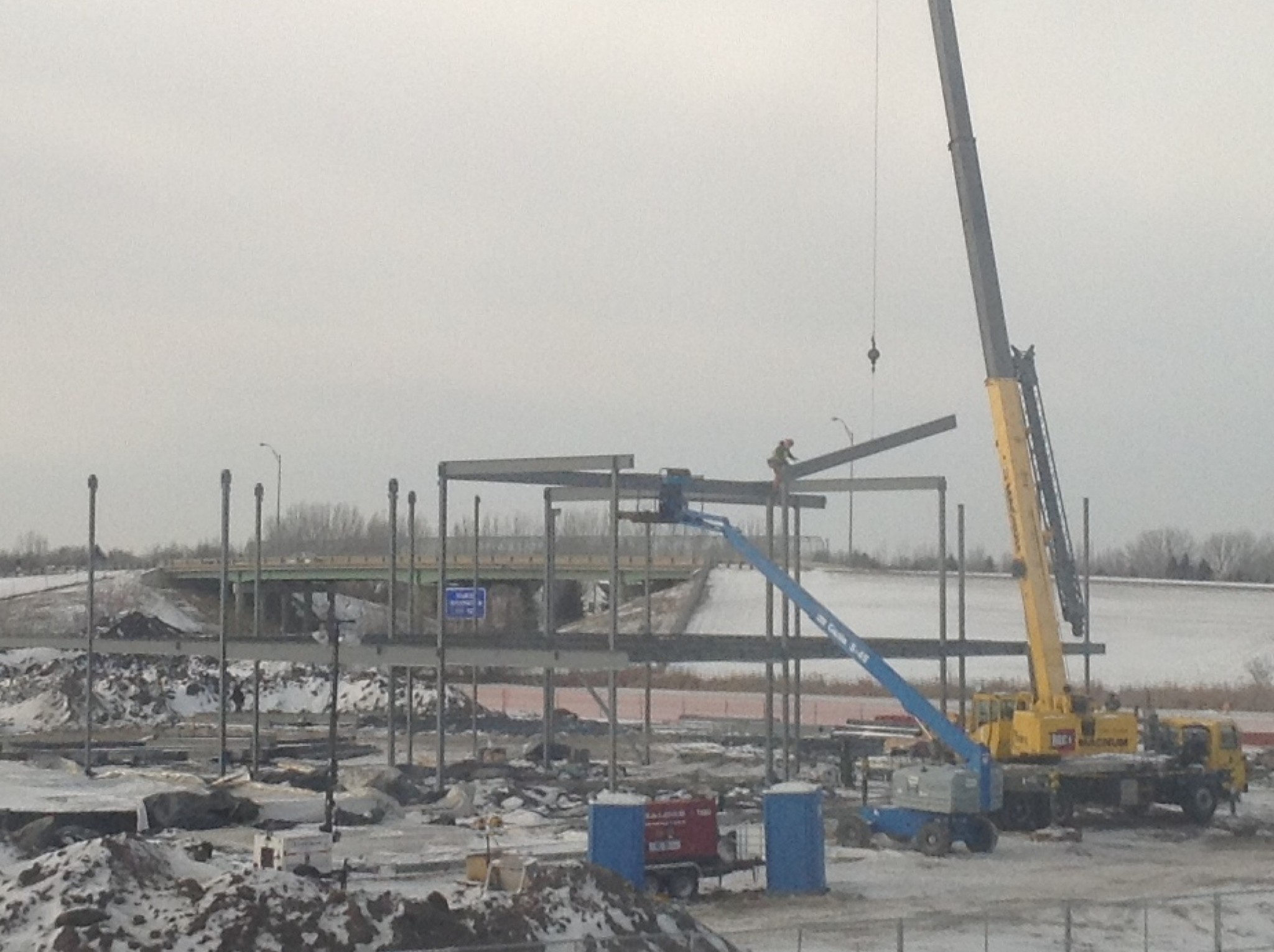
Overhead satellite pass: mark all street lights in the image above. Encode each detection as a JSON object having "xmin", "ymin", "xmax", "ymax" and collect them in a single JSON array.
[
  {"xmin": 259, "ymin": 443, "xmax": 282, "ymax": 532},
  {"xmin": 831, "ymin": 418, "xmax": 853, "ymax": 568}
]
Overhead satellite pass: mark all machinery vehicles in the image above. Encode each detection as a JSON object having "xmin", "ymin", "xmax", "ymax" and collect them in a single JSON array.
[
  {"xmin": 869, "ymin": 2, "xmax": 1248, "ymax": 836},
  {"xmin": 615, "ymin": 467, "xmax": 1004, "ymax": 857}
]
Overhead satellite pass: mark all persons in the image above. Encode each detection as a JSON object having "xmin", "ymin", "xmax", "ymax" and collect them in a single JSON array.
[
  {"xmin": 767, "ymin": 439, "xmax": 797, "ymax": 488},
  {"xmin": 1063, "ymin": 685, "xmax": 1094, "ymax": 736},
  {"xmin": 229, "ymin": 680, "xmax": 244, "ymax": 713},
  {"xmin": 1046, "ymin": 772, "xmax": 1060, "ymax": 830}
]
